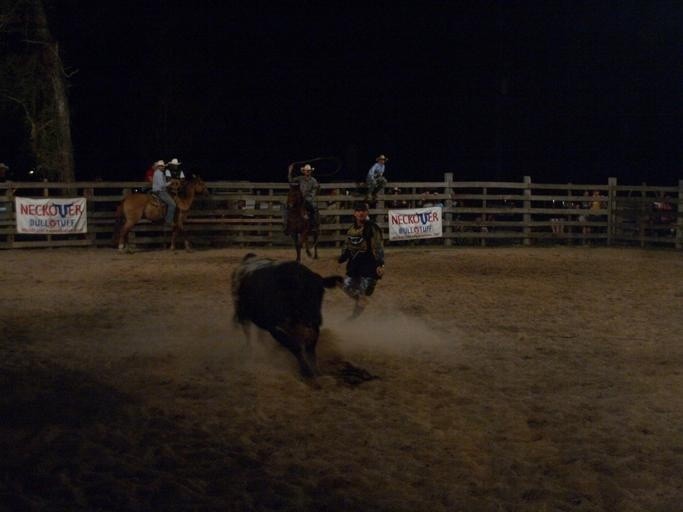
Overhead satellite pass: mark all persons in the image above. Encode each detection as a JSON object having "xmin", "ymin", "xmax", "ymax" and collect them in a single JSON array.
[
  {"xmin": 0, "ymin": 162, "xmax": 19, "ymax": 211},
  {"xmin": 331, "ymin": 200, "xmax": 389, "ymax": 320},
  {"xmin": 143, "ymin": 155, "xmax": 185, "ymax": 228},
  {"xmin": 552, "ymin": 190, "xmax": 681, "ymax": 244},
  {"xmin": 328, "ymin": 188, "xmax": 521, "ymax": 246},
  {"xmin": 281, "ymin": 160, "xmax": 320, "ymax": 236},
  {"xmin": 364, "ymin": 153, "xmax": 390, "ymax": 197}
]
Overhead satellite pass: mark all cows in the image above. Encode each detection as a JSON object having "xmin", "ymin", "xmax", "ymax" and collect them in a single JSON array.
[{"xmin": 228, "ymin": 252, "xmax": 345, "ymax": 392}]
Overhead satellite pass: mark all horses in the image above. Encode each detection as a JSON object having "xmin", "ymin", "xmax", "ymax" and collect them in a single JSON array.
[
  {"xmin": 284, "ymin": 181, "xmax": 318, "ymax": 262},
  {"xmin": 111, "ymin": 173, "xmax": 211, "ymax": 252}
]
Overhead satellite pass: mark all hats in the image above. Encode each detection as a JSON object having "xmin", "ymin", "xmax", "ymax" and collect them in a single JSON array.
[
  {"xmin": 300, "ymin": 164, "xmax": 315, "ymax": 173},
  {"xmin": 168, "ymin": 158, "xmax": 181, "ymax": 166},
  {"xmin": 375, "ymin": 155, "xmax": 388, "ymax": 161},
  {"xmin": 152, "ymin": 160, "xmax": 169, "ymax": 170}
]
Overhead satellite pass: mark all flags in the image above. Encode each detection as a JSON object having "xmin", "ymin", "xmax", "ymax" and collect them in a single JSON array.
[{"xmin": 14, "ymin": 197, "xmax": 88, "ymax": 237}]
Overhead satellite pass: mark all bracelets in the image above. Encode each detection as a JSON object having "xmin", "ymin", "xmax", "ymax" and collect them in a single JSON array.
[{"xmin": 378, "ymin": 263, "xmax": 385, "ymax": 268}]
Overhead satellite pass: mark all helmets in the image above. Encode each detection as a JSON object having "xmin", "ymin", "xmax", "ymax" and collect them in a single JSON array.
[{"xmin": 352, "ymin": 201, "xmax": 368, "ymax": 211}]
